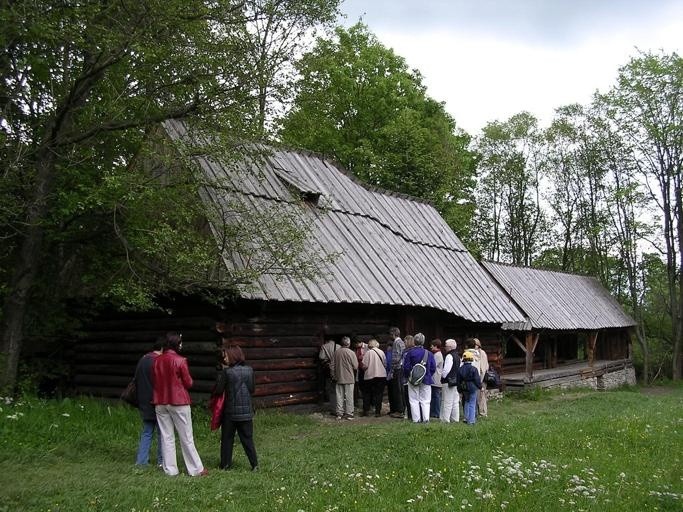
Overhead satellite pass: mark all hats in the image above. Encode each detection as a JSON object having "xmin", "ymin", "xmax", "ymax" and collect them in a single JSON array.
[{"xmin": 461, "ymin": 352, "xmax": 474, "ymax": 361}]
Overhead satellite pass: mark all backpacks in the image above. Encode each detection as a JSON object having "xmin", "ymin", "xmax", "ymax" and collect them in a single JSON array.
[
  {"xmin": 456, "ymin": 379, "xmax": 467, "ymax": 393},
  {"xmin": 120, "ymin": 378, "xmax": 139, "ymax": 409},
  {"xmin": 409, "ymin": 363, "xmax": 426, "ymax": 386},
  {"xmin": 485, "ymin": 368, "xmax": 501, "ymax": 389}
]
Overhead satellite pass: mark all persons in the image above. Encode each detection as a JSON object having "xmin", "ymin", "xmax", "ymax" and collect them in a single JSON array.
[
  {"xmin": 151, "ymin": 331, "xmax": 208, "ymax": 476},
  {"xmin": 211, "ymin": 344, "xmax": 259, "ymax": 473},
  {"xmin": 134, "ymin": 336, "xmax": 166, "ymax": 472},
  {"xmin": 318, "ymin": 326, "xmax": 490, "ymax": 424}
]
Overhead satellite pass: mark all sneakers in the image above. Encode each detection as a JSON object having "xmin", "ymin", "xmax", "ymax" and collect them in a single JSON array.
[
  {"xmin": 390, "ymin": 412, "xmax": 404, "ymax": 419},
  {"xmin": 335, "ymin": 416, "xmax": 342, "ymax": 420},
  {"xmin": 360, "ymin": 413, "xmax": 371, "ymax": 418},
  {"xmin": 374, "ymin": 413, "xmax": 382, "ymax": 418},
  {"xmin": 200, "ymin": 466, "xmax": 209, "ymax": 476},
  {"xmin": 345, "ymin": 414, "xmax": 355, "ymax": 420}
]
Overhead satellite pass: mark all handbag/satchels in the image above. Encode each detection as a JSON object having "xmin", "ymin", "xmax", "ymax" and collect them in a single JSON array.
[
  {"xmin": 448, "ymin": 378, "xmax": 458, "ymax": 388},
  {"xmin": 393, "ymin": 362, "xmax": 405, "ymax": 379},
  {"xmin": 211, "ymin": 392, "xmax": 225, "ymax": 432}
]
